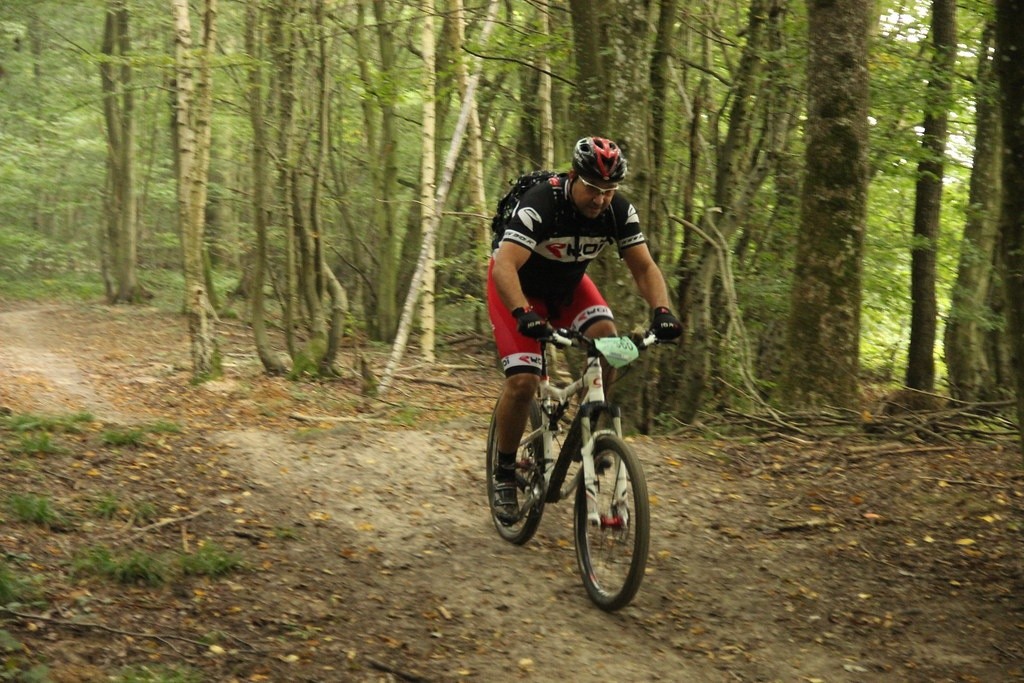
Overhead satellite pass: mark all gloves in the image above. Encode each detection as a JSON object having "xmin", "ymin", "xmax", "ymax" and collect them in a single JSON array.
[
  {"xmin": 512, "ymin": 305, "xmax": 554, "ymax": 341},
  {"xmin": 645, "ymin": 307, "xmax": 682, "ymax": 341}
]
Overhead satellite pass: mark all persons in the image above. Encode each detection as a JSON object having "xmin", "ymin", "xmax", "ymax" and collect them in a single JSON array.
[{"xmin": 486, "ymin": 135, "xmax": 684, "ymax": 525}]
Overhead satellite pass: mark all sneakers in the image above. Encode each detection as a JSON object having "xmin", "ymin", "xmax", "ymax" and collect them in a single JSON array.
[
  {"xmin": 594, "ymin": 451, "xmax": 614, "ymax": 472},
  {"xmin": 491, "ymin": 467, "xmax": 520, "ymax": 523}
]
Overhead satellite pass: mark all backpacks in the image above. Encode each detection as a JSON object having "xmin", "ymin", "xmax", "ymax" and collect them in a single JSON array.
[{"xmin": 491, "ymin": 164, "xmax": 616, "ymax": 249}]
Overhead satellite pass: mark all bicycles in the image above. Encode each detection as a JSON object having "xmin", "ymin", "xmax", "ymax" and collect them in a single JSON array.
[{"xmin": 486, "ymin": 306, "xmax": 678, "ymax": 614}]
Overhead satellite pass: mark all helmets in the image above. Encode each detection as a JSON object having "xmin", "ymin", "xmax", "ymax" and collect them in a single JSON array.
[{"xmin": 573, "ymin": 136, "xmax": 627, "ymax": 185}]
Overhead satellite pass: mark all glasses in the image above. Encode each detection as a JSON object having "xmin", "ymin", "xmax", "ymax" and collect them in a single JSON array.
[{"xmin": 578, "ymin": 173, "xmax": 619, "ymax": 197}]
